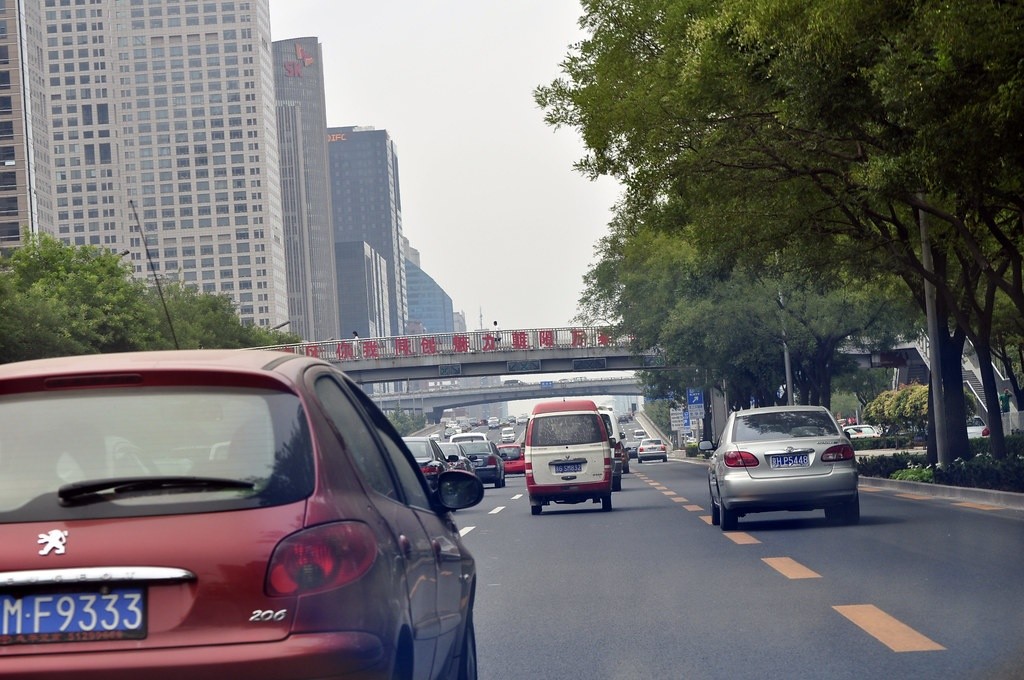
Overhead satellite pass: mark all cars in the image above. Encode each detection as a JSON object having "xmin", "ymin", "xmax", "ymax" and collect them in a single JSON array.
[
  {"xmin": 617, "ymin": 415, "xmax": 629, "ymax": 423},
  {"xmin": 0, "ymin": 348, "xmax": 486, "ymax": 680},
  {"xmin": 437, "ymin": 443, "xmax": 477, "ymax": 476},
  {"xmin": 448, "ymin": 433, "xmax": 489, "ymax": 442},
  {"xmin": 621, "ymin": 442, "xmax": 631, "ymax": 474},
  {"xmin": 598, "ymin": 409, "xmax": 625, "ymax": 491},
  {"xmin": 496, "ymin": 444, "xmax": 525, "ymax": 474},
  {"xmin": 623, "ymin": 413, "xmax": 634, "ymax": 421},
  {"xmin": 429, "ymin": 415, "xmax": 529, "ymax": 444},
  {"xmin": 624, "ymin": 442, "xmax": 641, "ymax": 458},
  {"xmin": 401, "ymin": 437, "xmax": 460, "ymax": 513},
  {"xmin": 632, "ymin": 430, "xmax": 647, "ymax": 442},
  {"xmin": 457, "ymin": 441, "xmax": 507, "ymax": 488},
  {"xmin": 966, "ymin": 415, "xmax": 990, "ymax": 438},
  {"xmin": 698, "ymin": 406, "xmax": 860, "ymax": 531},
  {"xmin": 842, "ymin": 425, "xmax": 881, "ymax": 438},
  {"xmin": 633, "ymin": 438, "xmax": 668, "ymax": 463}
]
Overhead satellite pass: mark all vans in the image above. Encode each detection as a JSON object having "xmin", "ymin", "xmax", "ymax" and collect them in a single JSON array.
[{"xmin": 524, "ymin": 400, "xmax": 616, "ymax": 515}]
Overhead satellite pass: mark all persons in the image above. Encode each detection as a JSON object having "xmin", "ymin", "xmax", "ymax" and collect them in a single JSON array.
[
  {"xmin": 352, "ymin": 331, "xmax": 360, "ymax": 358},
  {"xmin": 999, "ymin": 388, "xmax": 1013, "ymax": 412},
  {"xmin": 493, "ymin": 321, "xmax": 501, "ymax": 351}
]
